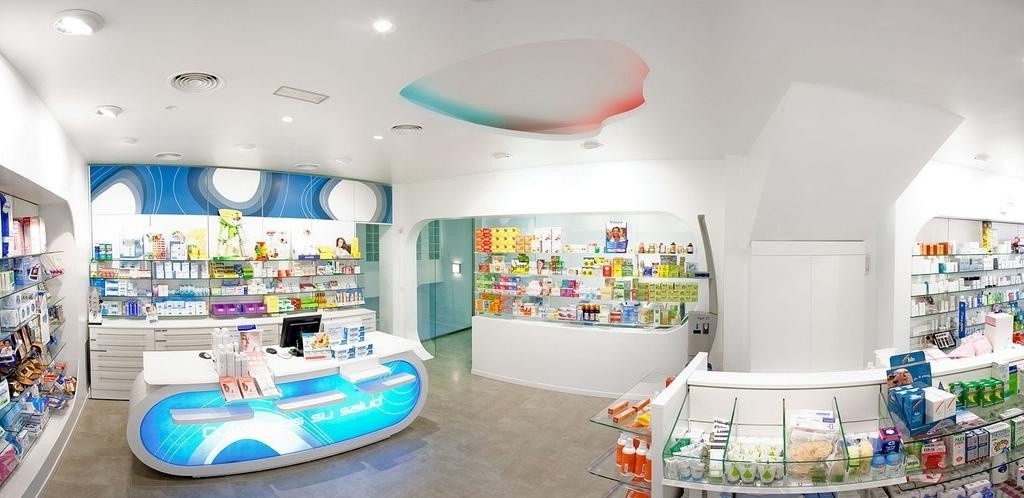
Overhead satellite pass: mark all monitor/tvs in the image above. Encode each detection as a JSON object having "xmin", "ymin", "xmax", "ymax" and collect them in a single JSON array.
[{"xmin": 279, "ymin": 312, "xmax": 323, "ymax": 357}]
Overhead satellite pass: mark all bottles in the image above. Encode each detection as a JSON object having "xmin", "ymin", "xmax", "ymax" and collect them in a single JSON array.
[
  {"xmin": 1001, "ymin": 304, "xmax": 1015, "ymax": 313},
  {"xmin": 960, "ymin": 296, "xmax": 977, "ymax": 308},
  {"xmin": 615, "ymin": 431, "xmax": 651, "ymax": 483},
  {"xmin": 640, "ymin": 240, "xmax": 693, "ymax": 255},
  {"xmin": 213, "ymin": 327, "xmax": 234, "ymax": 354},
  {"xmin": 873, "ymin": 451, "xmax": 901, "ymax": 478},
  {"xmin": 584, "ymin": 304, "xmax": 600, "ymax": 321},
  {"xmin": 94, "ymin": 243, "xmax": 99, "ymax": 261},
  {"xmin": 336, "ymin": 290, "xmax": 363, "ymax": 302}
]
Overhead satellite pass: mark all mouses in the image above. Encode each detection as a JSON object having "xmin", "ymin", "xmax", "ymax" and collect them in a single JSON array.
[
  {"xmin": 198, "ymin": 352, "xmax": 212, "ymax": 358},
  {"xmin": 265, "ymin": 347, "xmax": 276, "ymax": 355}
]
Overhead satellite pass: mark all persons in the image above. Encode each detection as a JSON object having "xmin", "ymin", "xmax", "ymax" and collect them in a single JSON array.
[
  {"xmin": 13, "ymin": 330, "xmax": 23, "ymax": 350},
  {"xmin": 612, "ymin": 226, "xmax": 620, "ymax": 241},
  {"xmin": 1, "ymin": 339, "xmax": 13, "ymax": 353},
  {"xmin": 335, "ymin": 236, "xmax": 350, "ymax": 254},
  {"xmin": 618, "ymin": 228, "xmax": 626, "ymax": 241},
  {"xmin": 310, "ymin": 333, "xmax": 330, "ymax": 350},
  {"xmin": 606, "ymin": 228, "xmax": 613, "ymax": 242},
  {"xmin": 245, "ymin": 334, "xmax": 260, "ymax": 352}
]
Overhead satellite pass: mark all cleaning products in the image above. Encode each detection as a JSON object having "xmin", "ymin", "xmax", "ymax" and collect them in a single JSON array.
[{"xmin": 613, "ymin": 431, "xmax": 652, "ymax": 498}]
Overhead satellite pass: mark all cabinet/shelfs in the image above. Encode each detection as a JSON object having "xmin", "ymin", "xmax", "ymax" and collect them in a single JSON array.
[
  {"xmin": 473, "ymin": 218, "xmax": 699, "ymax": 328},
  {"xmin": 0, "ymin": 166, "xmax": 86, "ymax": 498},
  {"xmin": 907, "ymin": 214, "xmax": 1024, "ymax": 361}
]
